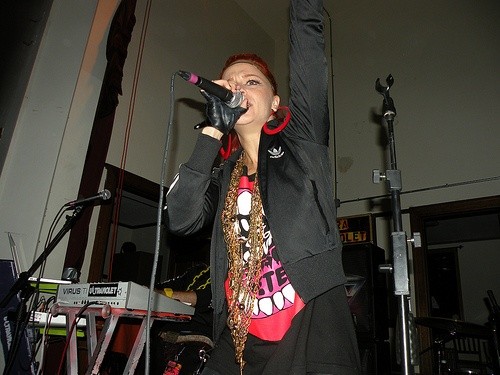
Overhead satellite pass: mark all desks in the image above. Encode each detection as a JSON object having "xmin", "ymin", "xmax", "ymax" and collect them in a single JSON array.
[
  {"xmin": 43, "ymin": 304, "xmax": 190, "ymax": 375},
  {"xmin": 415, "ymin": 316, "xmax": 500, "ymax": 375}
]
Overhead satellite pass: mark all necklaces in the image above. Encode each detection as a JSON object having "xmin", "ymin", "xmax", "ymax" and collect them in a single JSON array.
[{"xmin": 220, "ymin": 152, "xmax": 264, "ymax": 368}]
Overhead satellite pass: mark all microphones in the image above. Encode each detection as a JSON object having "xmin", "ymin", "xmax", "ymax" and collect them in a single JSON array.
[
  {"xmin": 65, "ymin": 189, "xmax": 111, "ymax": 206},
  {"xmin": 176, "ymin": 69, "xmax": 244, "ymax": 108}
]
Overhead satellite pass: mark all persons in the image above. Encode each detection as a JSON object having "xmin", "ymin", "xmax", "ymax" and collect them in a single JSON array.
[
  {"xmin": 146, "ymin": 233, "xmax": 213, "ymax": 375},
  {"xmin": 167, "ymin": 0, "xmax": 366, "ymax": 375}
]
